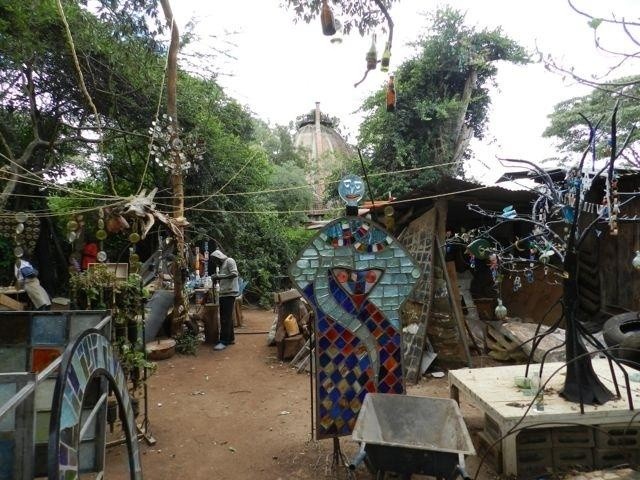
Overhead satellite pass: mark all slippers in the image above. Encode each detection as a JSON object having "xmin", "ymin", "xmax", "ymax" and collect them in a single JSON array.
[
  {"xmin": 213, "ymin": 343, "xmax": 228, "ymax": 351},
  {"xmin": 216, "ymin": 340, "xmax": 236, "ymax": 345}
]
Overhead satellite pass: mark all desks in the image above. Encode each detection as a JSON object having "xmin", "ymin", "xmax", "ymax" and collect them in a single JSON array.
[{"xmin": 447, "ymin": 360, "xmax": 640, "ymax": 479}]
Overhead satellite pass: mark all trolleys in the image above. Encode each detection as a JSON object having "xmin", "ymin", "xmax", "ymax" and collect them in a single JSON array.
[{"xmin": 348, "ymin": 391, "xmax": 479, "ymax": 480}]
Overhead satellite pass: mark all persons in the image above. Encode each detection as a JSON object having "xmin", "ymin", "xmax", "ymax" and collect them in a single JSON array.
[
  {"xmin": 210, "ymin": 249, "xmax": 240, "ymax": 351},
  {"xmin": 82, "ymin": 234, "xmax": 98, "ymax": 270},
  {"xmin": 13, "ymin": 243, "xmax": 52, "ymax": 311}
]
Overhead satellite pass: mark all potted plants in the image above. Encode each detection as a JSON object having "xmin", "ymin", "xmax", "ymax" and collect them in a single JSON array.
[{"xmin": 72, "ymin": 264, "xmax": 152, "ymax": 434}]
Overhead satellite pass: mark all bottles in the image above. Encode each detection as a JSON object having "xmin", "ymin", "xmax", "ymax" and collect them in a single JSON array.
[
  {"xmin": 215, "ymin": 280, "xmax": 220, "ymax": 290},
  {"xmin": 530, "ymin": 371, "xmax": 544, "ymax": 411}
]
